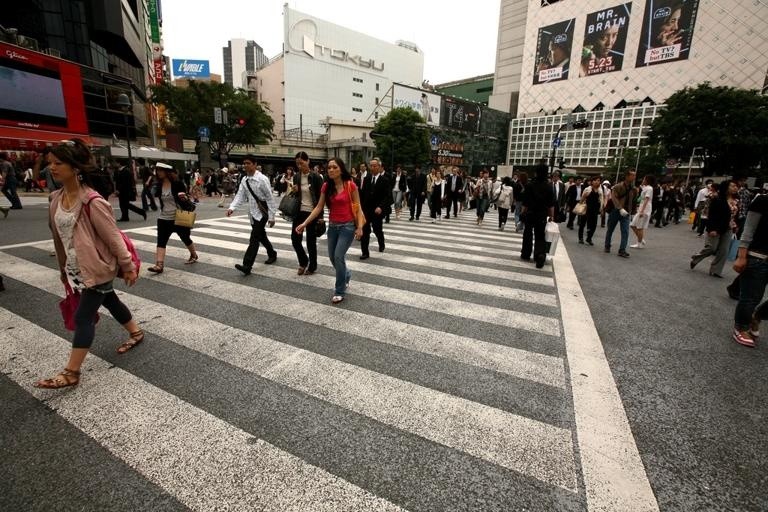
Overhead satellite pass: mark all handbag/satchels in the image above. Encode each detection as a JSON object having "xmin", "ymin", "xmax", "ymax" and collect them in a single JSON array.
[
  {"xmin": 314, "ymin": 220, "xmax": 326, "ymax": 237},
  {"xmin": 173, "ymin": 206, "xmax": 196, "ymax": 227},
  {"xmin": 572, "ymin": 197, "xmax": 588, "ymax": 214},
  {"xmin": 60, "ymin": 281, "xmax": 101, "ymax": 332},
  {"xmin": 278, "ymin": 191, "xmax": 299, "ymax": 219}
]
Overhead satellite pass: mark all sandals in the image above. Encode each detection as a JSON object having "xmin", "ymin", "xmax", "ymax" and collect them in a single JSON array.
[
  {"xmin": 185, "ymin": 255, "xmax": 198, "ymax": 264},
  {"xmin": 332, "ymin": 295, "xmax": 343, "ymax": 302},
  {"xmin": 31, "ymin": 369, "xmax": 82, "ymax": 388},
  {"xmin": 148, "ymin": 263, "xmax": 164, "ymax": 272},
  {"xmin": 345, "ymin": 279, "xmax": 351, "ymax": 289},
  {"xmin": 117, "ymin": 330, "xmax": 144, "ymax": 354}
]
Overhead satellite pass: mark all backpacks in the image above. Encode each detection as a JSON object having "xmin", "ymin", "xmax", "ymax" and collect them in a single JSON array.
[{"xmin": 82, "ymin": 189, "xmax": 141, "ymax": 279}]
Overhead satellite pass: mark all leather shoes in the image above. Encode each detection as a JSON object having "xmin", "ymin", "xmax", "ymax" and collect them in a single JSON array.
[
  {"xmin": 378, "ymin": 245, "xmax": 385, "ymax": 252},
  {"xmin": 116, "ymin": 218, "xmax": 130, "ymax": 222},
  {"xmin": 235, "ymin": 264, "xmax": 251, "ymax": 276},
  {"xmin": 416, "ymin": 217, "xmax": 419, "ymax": 220},
  {"xmin": 409, "ymin": 217, "xmax": 414, "ymax": 221},
  {"xmin": 710, "ymin": 272, "xmax": 724, "ymax": 278},
  {"xmin": 690, "ymin": 255, "xmax": 700, "ymax": 269},
  {"xmin": 360, "ymin": 255, "xmax": 369, "ymax": 260},
  {"xmin": 264, "ymin": 255, "xmax": 278, "ymax": 265},
  {"xmin": 140, "ymin": 208, "xmax": 147, "ymax": 221}
]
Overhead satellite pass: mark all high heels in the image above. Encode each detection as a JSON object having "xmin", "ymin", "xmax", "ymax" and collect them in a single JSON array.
[
  {"xmin": 297, "ymin": 266, "xmax": 305, "ymax": 275},
  {"xmin": 304, "ymin": 270, "xmax": 313, "ymax": 275}
]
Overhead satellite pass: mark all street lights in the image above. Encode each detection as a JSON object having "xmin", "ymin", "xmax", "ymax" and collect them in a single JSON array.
[{"xmin": 116, "ymin": 94, "xmax": 133, "ymax": 170}]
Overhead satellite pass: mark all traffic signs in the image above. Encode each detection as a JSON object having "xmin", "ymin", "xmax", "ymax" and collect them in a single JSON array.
[
  {"xmin": 431, "ymin": 137, "xmax": 440, "ymax": 145},
  {"xmin": 552, "ymin": 138, "xmax": 561, "ymax": 148},
  {"xmin": 199, "ymin": 126, "xmax": 210, "ymax": 142}
]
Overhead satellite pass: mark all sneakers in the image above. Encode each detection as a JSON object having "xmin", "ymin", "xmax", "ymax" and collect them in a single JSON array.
[
  {"xmin": 536, "ymin": 259, "xmax": 544, "ymax": 268},
  {"xmin": 605, "ymin": 245, "xmax": 610, "ymax": 253},
  {"xmin": 731, "ymin": 325, "xmax": 755, "ymax": 347},
  {"xmin": 500, "ymin": 222, "xmax": 505, "ymax": 230},
  {"xmin": 586, "ymin": 239, "xmax": 594, "ymax": 246},
  {"xmin": 748, "ymin": 318, "xmax": 760, "ymax": 339},
  {"xmin": 520, "ymin": 255, "xmax": 531, "ymax": 262},
  {"xmin": 630, "ymin": 244, "xmax": 642, "ymax": 248},
  {"xmin": 642, "ymin": 240, "xmax": 647, "ymax": 245},
  {"xmin": 579, "ymin": 240, "xmax": 584, "ymax": 244},
  {"xmin": 617, "ymin": 250, "xmax": 630, "ymax": 257}
]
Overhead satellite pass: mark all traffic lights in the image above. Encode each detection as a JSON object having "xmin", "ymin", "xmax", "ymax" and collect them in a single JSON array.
[
  {"xmin": 573, "ymin": 119, "xmax": 590, "ymax": 129},
  {"xmin": 230, "ymin": 116, "xmax": 246, "ymax": 127}
]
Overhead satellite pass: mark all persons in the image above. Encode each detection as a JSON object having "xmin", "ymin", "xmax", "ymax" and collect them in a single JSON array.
[
  {"xmin": 112, "ymin": 157, "xmax": 147, "ymax": 222},
  {"xmin": 511, "ymin": 174, "xmax": 519, "ymax": 213},
  {"xmin": 603, "ymin": 169, "xmax": 638, "ymax": 257},
  {"xmin": 274, "ymin": 171, "xmax": 284, "ymax": 196},
  {"xmin": 533, "ymin": 32, "xmax": 571, "ymax": 85},
  {"xmin": 280, "ymin": 167, "xmax": 294, "ymax": 194},
  {"xmin": 426, "ymin": 167, "xmax": 441, "ymax": 218},
  {"xmin": 24, "ymin": 164, "xmax": 33, "ymax": 192},
  {"xmin": 565, "ymin": 176, "xmax": 586, "ymax": 229},
  {"xmin": 627, "ymin": 174, "xmax": 655, "ymax": 250},
  {"xmin": 313, "ymin": 165, "xmax": 323, "ymax": 180},
  {"xmin": 512, "ymin": 173, "xmax": 528, "ymax": 233},
  {"xmin": 444, "ymin": 169, "xmax": 463, "ymax": 219},
  {"xmin": 278, "ymin": 152, "xmax": 325, "ymax": 276},
  {"xmin": 32, "ymin": 146, "xmax": 62, "ymax": 193},
  {"xmin": 662, "ymin": 181, "xmax": 702, "ymax": 227},
  {"xmin": 147, "ymin": 161, "xmax": 198, "ymax": 276},
  {"xmin": 518, "ymin": 164, "xmax": 560, "ymax": 269},
  {"xmin": 0, "ymin": 152, "xmax": 23, "ymax": 209},
  {"xmin": 696, "ymin": 183, "xmax": 720, "ymax": 238},
  {"xmin": 687, "ymin": 179, "xmax": 713, "ymax": 230},
  {"xmin": 470, "ymin": 168, "xmax": 497, "ymax": 225},
  {"xmin": 350, "ymin": 167, "xmax": 359, "ymax": 184},
  {"xmin": 732, "ymin": 171, "xmax": 752, "ymax": 220},
  {"xmin": 9, "ymin": 152, "xmax": 22, "ymax": 181},
  {"xmin": 359, "ymin": 158, "xmax": 392, "ymax": 260},
  {"xmin": 226, "ymin": 155, "xmax": 277, "ymax": 276},
  {"xmin": 459, "ymin": 171, "xmax": 476, "ymax": 213},
  {"xmin": 750, "ymin": 188, "xmax": 768, "ymax": 204},
  {"xmin": 564, "ymin": 176, "xmax": 576, "ymax": 195},
  {"xmin": 302, "ymin": 176, "xmax": 374, "ymax": 323},
  {"xmin": 136, "ymin": 157, "xmax": 158, "ymax": 212},
  {"xmin": 34, "ymin": 138, "xmax": 145, "ymax": 391},
  {"xmin": 0, "ymin": 207, "xmax": 10, "ymax": 218},
  {"xmin": 578, "ymin": 10, "xmax": 623, "ymax": 76},
  {"xmin": 726, "ymin": 195, "xmax": 768, "ymax": 348},
  {"xmin": 649, "ymin": 181, "xmax": 669, "ymax": 229},
  {"xmin": 356, "ymin": 161, "xmax": 368, "ymax": 190},
  {"xmin": 380, "ymin": 162, "xmax": 394, "ymax": 223},
  {"xmin": 477, "ymin": 171, "xmax": 484, "ymax": 185},
  {"xmin": 217, "ymin": 167, "xmax": 239, "ymax": 209},
  {"xmin": 572, "ymin": 176, "xmax": 604, "ymax": 246},
  {"xmin": 642, "ymin": 0, "xmax": 684, "ymax": 52},
  {"xmin": 491, "ymin": 176, "xmax": 514, "ymax": 231},
  {"xmin": 204, "ymin": 168, "xmax": 222, "ymax": 197},
  {"xmin": 553, "ymin": 170, "xmax": 566, "ymax": 224},
  {"xmin": 406, "ymin": 164, "xmax": 427, "ymax": 222},
  {"xmin": 189, "ymin": 168, "xmax": 204, "ymax": 203},
  {"xmin": 601, "ymin": 180, "xmax": 612, "ymax": 227},
  {"xmin": 430, "ymin": 169, "xmax": 446, "ymax": 223},
  {"xmin": 690, "ymin": 180, "xmax": 739, "ymax": 278},
  {"xmin": 389, "ymin": 164, "xmax": 407, "ymax": 214}
]
